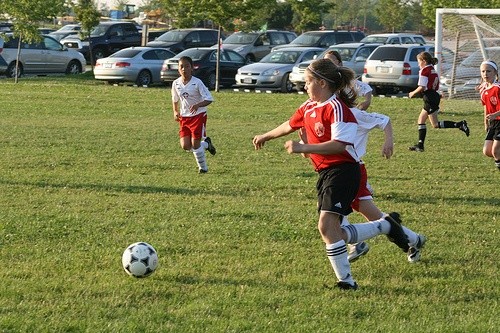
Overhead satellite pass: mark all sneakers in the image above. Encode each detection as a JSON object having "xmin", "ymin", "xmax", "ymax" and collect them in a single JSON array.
[
  {"xmin": 204, "ymin": 136, "xmax": 216, "ymax": 156},
  {"xmin": 407, "ymin": 233, "xmax": 427, "ymax": 263},
  {"xmin": 459, "ymin": 120, "xmax": 470, "ymax": 137},
  {"xmin": 347, "ymin": 241, "xmax": 369, "ymax": 262},
  {"xmin": 408, "ymin": 144, "xmax": 424, "ymax": 152},
  {"xmin": 198, "ymin": 168, "xmax": 208, "ymax": 174},
  {"xmin": 333, "ymin": 281, "xmax": 359, "ymax": 291},
  {"xmin": 384, "ymin": 212, "xmax": 410, "ymax": 253}
]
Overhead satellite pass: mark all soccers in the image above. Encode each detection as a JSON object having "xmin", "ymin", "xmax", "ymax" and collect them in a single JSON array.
[{"xmin": 122, "ymin": 242, "xmax": 159, "ymax": 278}]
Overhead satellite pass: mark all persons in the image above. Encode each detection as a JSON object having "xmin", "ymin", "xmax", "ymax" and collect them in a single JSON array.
[
  {"xmin": 172, "ymin": 57, "xmax": 216, "ymax": 175},
  {"xmin": 409, "ymin": 51, "xmax": 471, "ymax": 152},
  {"xmin": 478, "ymin": 60, "xmax": 500, "ymax": 172},
  {"xmin": 252, "ymin": 51, "xmax": 427, "ymax": 291}
]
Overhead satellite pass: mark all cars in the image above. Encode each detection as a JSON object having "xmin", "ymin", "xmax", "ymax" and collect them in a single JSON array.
[{"xmin": 1, "ymin": 21, "xmax": 500, "ymax": 98}]
